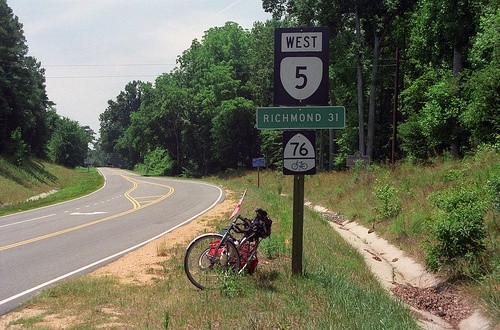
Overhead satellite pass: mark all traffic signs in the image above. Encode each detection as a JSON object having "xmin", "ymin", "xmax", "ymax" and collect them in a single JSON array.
[{"xmin": 273, "ymin": 28, "xmax": 330, "ymax": 106}]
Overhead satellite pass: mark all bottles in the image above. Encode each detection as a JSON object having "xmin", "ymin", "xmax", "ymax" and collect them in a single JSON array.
[{"xmin": 220, "ymin": 251, "xmax": 228, "ymax": 266}]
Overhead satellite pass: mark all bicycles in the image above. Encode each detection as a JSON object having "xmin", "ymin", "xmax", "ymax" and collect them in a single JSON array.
[{"xmin": 184, "ymin": 207, "xmax": 274, "ymax": 291}]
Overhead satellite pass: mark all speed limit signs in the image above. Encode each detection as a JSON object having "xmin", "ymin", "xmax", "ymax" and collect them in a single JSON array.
[{"xmin": 282, "ymin": 129, "xmax": 317, "ymax": 176}]
